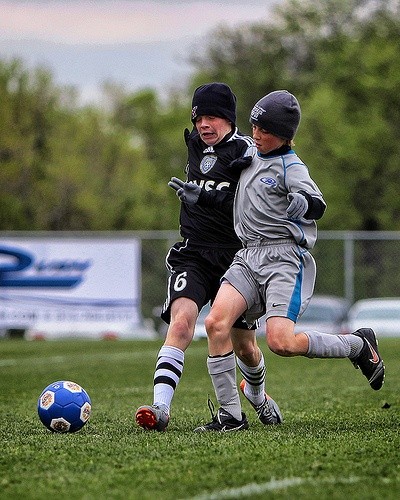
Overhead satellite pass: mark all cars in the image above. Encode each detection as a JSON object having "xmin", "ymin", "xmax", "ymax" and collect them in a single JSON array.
[
  {"xmin": 340, "ymin": 297, "xmax": 400, "ymax": 338},
  {"xmin": 152, "ymin": 300, "xmax": 213, "ymax": 341},
  {"xmin": 24, "ymin": 314, "xmax": 158, "ymax": 342},
  {"xmin": 260, "ymin": 296, "xmax": 346, "ymax": 339}
]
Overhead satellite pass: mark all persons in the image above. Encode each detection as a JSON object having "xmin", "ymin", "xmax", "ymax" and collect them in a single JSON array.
[
  {"xmin": 134, "ymin": 83, "xmax": 282, "ymax": 428},
  {"xmin": 167, "ymin": 91, "xmax": 386, "ymax": 431}
]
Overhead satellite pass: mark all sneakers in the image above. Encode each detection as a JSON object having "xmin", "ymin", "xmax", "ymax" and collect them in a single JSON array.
[
  {"xmin": 193, "ymin": 399, "xmax": 250, "ymax": 435},
  {"xmin": 347, "ymin": 327, "xmax": 386, "ymax": 392},
  {"xmin": 238, "ymin": 377, "xmax": 282, "ymax": 425},
  {"xmin": 135, "ymin": 401, "xmax": 171, "ymax": 432}
]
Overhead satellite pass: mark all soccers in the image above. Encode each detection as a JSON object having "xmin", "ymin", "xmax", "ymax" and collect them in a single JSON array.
[{"xmin": 36, "ymin": 380, "xmax": 93, "ymax": 435}]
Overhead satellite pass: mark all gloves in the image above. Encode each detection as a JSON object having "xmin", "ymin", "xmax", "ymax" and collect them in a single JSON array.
[
  {"xmin": 168, "ymin": 174, "xmax": 203, "ymax": 207},
  {"xmin": 283, "ymin": 191, "xmax": 309, "ymax": 222}
]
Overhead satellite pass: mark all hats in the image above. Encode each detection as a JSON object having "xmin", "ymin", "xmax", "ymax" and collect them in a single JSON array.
[
  {"xmin": 248, "ymin": 89, "xmax": 302, "ymax": 141},
  {"xmin": 189, "ymin": 82, "xmax": 239, "ymax": 125}
]
